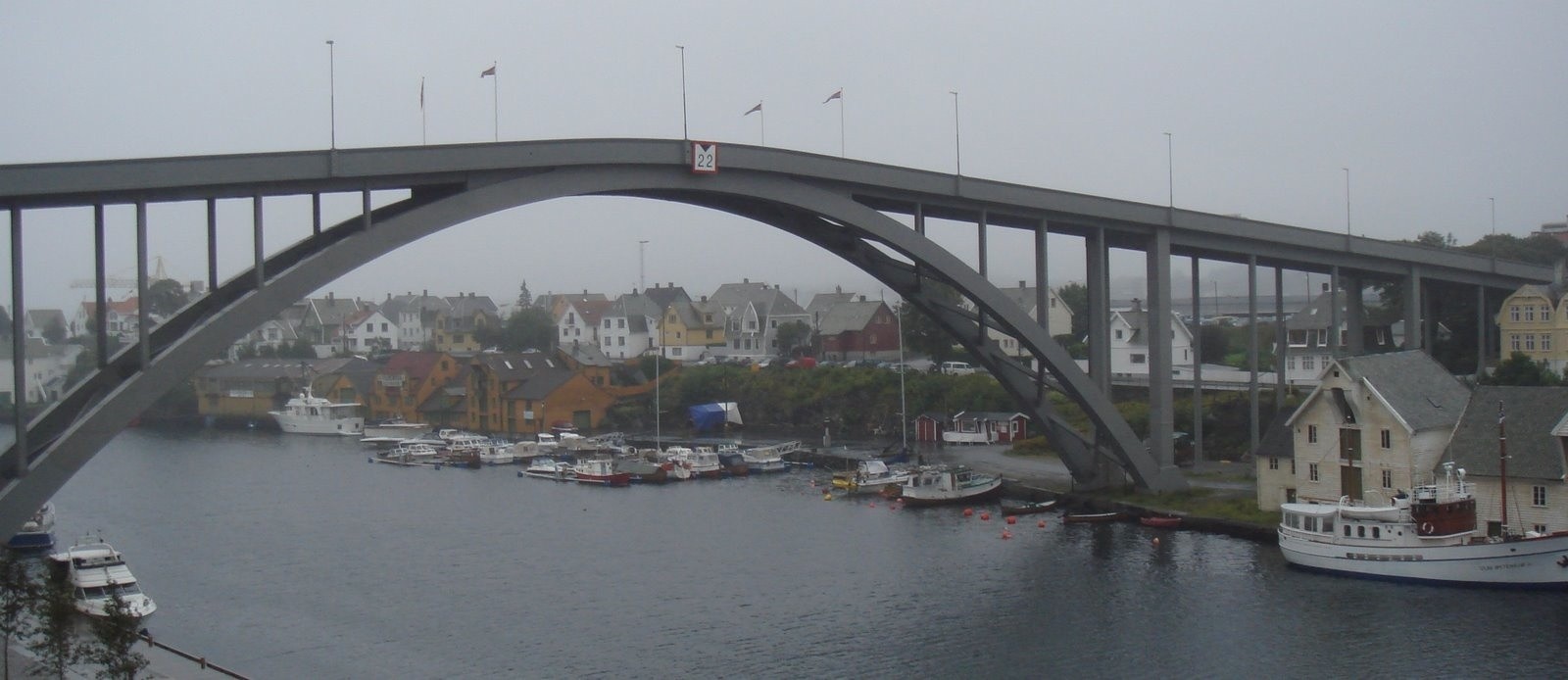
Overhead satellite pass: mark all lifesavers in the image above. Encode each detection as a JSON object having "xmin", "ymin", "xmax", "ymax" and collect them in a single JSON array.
[{"xmin": 1422, "ymin": 522, "xmax": 1434, "ymax": 534}]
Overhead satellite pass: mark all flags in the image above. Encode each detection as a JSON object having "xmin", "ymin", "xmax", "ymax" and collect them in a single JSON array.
[
  {"xmin": 480, "ymin": 65, "xmax": 496, "ymax": 79},
  {"xmin": 822, "ymin": 90, "xmax": 840, "ymax": 104},
  {"xmin": 743, "ymin": 104, "xmax": 761, "ymax": 116}
]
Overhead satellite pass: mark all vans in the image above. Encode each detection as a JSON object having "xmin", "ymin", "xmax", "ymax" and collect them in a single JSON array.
[{"xmin": 941, "ymin": 361, "xmax": 977, "ymax": 378}]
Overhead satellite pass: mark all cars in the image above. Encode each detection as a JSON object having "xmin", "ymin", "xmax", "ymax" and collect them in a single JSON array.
[{"xmin": 697, "ymin": 354, "xmax": 919, "ymax": 375}]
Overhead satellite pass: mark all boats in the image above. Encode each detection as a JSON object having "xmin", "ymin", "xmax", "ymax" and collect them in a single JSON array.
[
  {"xmin": 43, "ymin": 529, "xmax": 158, "ymax": 626},
  {"xmin": 1275, "ymin": 398, "xmax": 1568, "ymax": 591},
  {"xmin": 1140, "ymin": 516, "xmax": 1182, "ymax": 529},
  {"xmin": 1056, "ymin": 508, "xmax": 1129, "ymax": 523},
  {"xmin": 999, "ymin": 499, "xmax": 1057, "ymax": 516},
  {"xmin": 7, "ymin": 502, "xmax": 58, "ymax": 554},
  {"xmin": 265, "ymin": 380, "xmax": 366, "ymax": 436},
  {"xmin": 900, "ymin": 463, "xmax": 1004, "ymax": 506},
  {"xmin": 360, "ymin": 347, "xmax": 786, "ymax": 487}
]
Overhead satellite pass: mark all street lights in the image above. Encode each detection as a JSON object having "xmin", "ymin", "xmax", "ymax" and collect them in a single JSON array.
[
  {"xmin": 1164, "ymin": 132, "xmax": 1173, "ymax": 207},
  {"xmin": 1343, "ymin": 168, "xmax": 1350, "ymax": 235},
  {"xmin": 1489, "ymin": 198, "xmax": 1496, "ymax": 260},
  {"xmin": 675, "ymin": 45, "xmax": 688, "ymax": 140},
  {"xmin": 949, "ymin": 90, "xmax": 961, "ymax": 175},
  {"xmin": 326, "ymin": 40, "xmax": 335, "ymax": 150}
]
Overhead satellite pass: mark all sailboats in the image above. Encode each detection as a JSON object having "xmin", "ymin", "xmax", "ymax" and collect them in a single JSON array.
[{"xmin": 832, "ymin": 307, "xmax": 932, "ymax": 494}]
